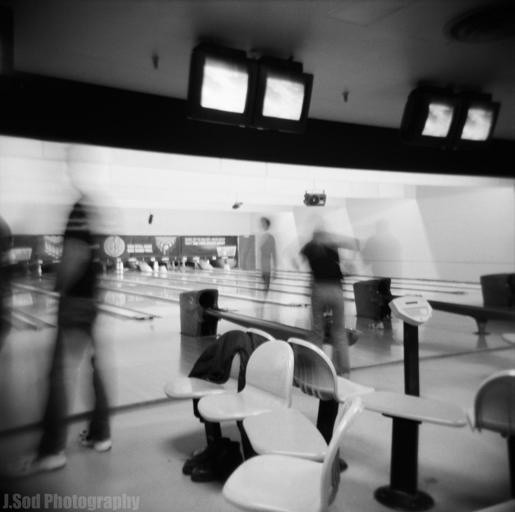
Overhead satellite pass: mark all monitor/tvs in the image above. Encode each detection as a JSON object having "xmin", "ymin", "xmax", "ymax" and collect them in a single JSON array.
[
  {"xmin": 400, "ymin": 86, "xmax": 501, "ymax": 148},
  {"xmin": 188, "ymin": 46, "xmax": 314, "ymax": 134}
]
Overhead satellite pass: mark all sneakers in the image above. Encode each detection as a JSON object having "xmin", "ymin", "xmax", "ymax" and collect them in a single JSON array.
[
  {"xmin": 183, "ymin": 436, "xmax": 243, "ymax": 483},
  {"xmin": 79, "ymin": 429, "xmax": 112, "ymax": 453},
  {"xmin": 16, "ymin": 451, "xmax": 67, "ymax": 475}
]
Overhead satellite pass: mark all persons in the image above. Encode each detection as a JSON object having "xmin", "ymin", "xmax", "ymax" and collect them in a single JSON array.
[
  {"xmin": 303, "ymin": 219, "xmax": 363, "ymax": 377},
  {"xmin": 236, "ymin": 211, "xmax": 283, "ymax": 324},
  {"xmin": 32, "ymin": 146, "xmax": 115, "ymax": 472},
  {"xmin": 359, "ymin": 215, "xmax": 401, "ymax": 336}
]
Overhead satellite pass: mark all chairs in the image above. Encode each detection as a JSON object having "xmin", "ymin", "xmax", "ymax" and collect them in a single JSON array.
[
  {"xmin": 465, "ymin": 371, "xmax": 515, "ymax": 499},
  {"xmin": 163, "ymin": 327, "xmax": 376, "ymax": 511}
]
